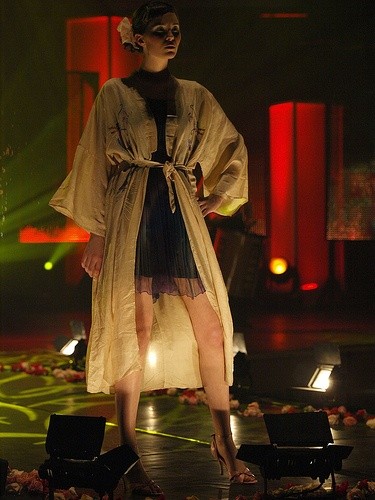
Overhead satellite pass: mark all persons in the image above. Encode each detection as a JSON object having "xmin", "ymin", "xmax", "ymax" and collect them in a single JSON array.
[{"xmin": 48, "ymin": 1, "xmax": 258, "ymax": 494}]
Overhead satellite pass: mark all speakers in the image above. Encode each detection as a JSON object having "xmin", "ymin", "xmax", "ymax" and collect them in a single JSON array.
[{"xmin": 219, "ymin": 229, "xmax": 268, "ymax": 301}]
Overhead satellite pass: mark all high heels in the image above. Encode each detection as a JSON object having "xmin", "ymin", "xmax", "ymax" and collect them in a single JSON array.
[
  {"xmin": 122, "ymin": 475, "xmax": 164, "ymax": 495},
  {"xmin": 211, "ymin": 433, "xmax": 258, "ymax": 483}
]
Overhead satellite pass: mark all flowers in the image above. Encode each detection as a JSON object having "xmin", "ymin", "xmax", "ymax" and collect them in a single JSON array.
[
  {"xmin": 3, "ymin": 350, "xmax": 375, "ymax": 432},
  {"xmin": 6, "ymin": 464, "xmax": 375, "ymax": 500}
]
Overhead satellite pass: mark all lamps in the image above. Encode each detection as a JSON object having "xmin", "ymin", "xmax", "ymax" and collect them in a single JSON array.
[
  {"xmin": 38, "ymin": 414, "xmax": 139, "ymax": 500},
  {"xmin": 258, "ymin": 256, "xmax": 300, "ymax": 297},
  {"xmin": 235, "ymin": 411, "xmax": 354, "ymax": 496}
]
